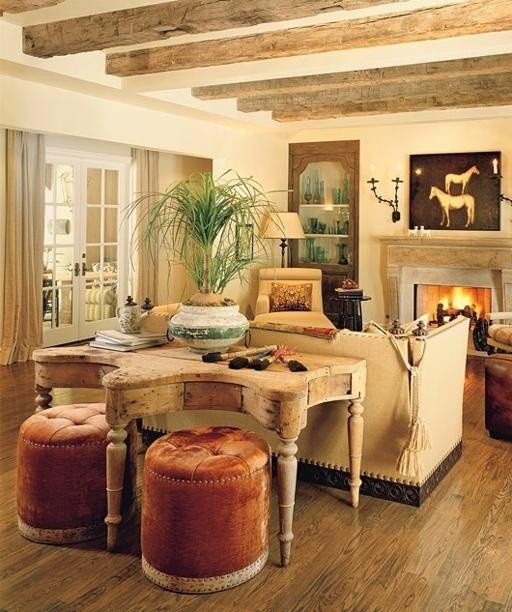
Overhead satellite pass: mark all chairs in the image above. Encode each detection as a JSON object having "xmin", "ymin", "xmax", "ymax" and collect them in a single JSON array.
[
  {"xmin": 256, "ymin": 266, "xmax": 338, "ymax": 330},
  {"xmin": 85, "ymin": 258, "xmax": 118, "ymax": 321}
]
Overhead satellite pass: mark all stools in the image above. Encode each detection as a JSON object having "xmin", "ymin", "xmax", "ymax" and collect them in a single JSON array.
[
  {"xmin": 16, "ymin": 401, "xmax": 140, "ymax": 545},
  {"xmin": 139, "ymin": 427, "xmax": 275, "ymax": 595}
]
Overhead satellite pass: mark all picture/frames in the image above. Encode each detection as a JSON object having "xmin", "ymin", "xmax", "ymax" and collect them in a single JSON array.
[
  {"xmin": 408, "ymin": 152, "xmax": 501, "ymax": 232},
  {"xmin": 235, "ymin": 224, "xmax": 254, "ymax": 263}
]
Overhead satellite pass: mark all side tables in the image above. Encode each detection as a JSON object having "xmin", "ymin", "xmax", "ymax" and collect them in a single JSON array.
[{"xmin": 328, "ymin": 296, "xmax": 372, "ymax": 331}]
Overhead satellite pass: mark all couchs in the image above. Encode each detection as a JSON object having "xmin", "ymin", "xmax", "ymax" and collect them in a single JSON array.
[{"xmin": 141, "ymin": 301, "xmax": 470, "ymax": 508}]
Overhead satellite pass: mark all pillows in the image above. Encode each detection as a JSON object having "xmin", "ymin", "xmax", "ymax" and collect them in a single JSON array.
[{"xmin": 269, "ymin": 278, "xmax": 312, "ymax": 311}]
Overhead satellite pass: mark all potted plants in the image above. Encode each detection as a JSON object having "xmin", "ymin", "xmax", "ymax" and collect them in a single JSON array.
[{"xmin": 121, "ymin": 167, "xmax": 291, "ymax": 355}]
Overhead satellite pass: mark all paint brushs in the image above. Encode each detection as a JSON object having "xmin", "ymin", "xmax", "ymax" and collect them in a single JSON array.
[{"xmin": 203, "ymin": 345, "xmax": 308, "ymax": 372}]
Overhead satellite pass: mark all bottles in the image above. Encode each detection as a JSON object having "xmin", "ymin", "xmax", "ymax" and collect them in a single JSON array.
[{"xmin": 302, "ymin": 168, "xmax": 349, "ymax": 265}]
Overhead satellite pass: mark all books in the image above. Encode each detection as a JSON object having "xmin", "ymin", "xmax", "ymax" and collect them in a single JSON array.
[{"xmin": 90, "ymin": 327, "xmax": 171, "ymax": 352}]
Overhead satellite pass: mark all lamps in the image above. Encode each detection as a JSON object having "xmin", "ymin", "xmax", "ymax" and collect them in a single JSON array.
[
  {"xmin": 260, "ymin": 212, "xmax": 305, "ymax": 267},
  {"xmin": 366, "ymin": 163, "xmax": 404, "ymax": 222}
]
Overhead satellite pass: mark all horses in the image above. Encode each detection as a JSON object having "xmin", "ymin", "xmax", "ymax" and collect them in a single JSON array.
[
  {"xmin": 443, "ymin": 164, "xmax": 479, "ymax": 195},
  {"xmin": 428, "ymin": 185, "xmax": 475, "ymax": 228}
]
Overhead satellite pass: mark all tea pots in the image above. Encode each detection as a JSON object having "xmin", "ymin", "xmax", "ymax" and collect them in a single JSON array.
[{"xmin": 115, "ymin": 296, "xmax": 150, "ymax": 334}]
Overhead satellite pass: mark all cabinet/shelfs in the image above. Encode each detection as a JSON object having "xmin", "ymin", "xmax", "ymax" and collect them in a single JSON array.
[{"xmin": 288, "ymin": 141, "xmax": 360, "ymax": 328}]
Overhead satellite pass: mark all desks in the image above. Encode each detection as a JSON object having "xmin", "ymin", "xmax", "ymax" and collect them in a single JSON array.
[
  {"xmin": 42, "ymin": 272, "xmax": 118, "ymax": 326},
  {"xmin": 31, "ymin": 341, "xmax": 369, "ymax": 565}
]
